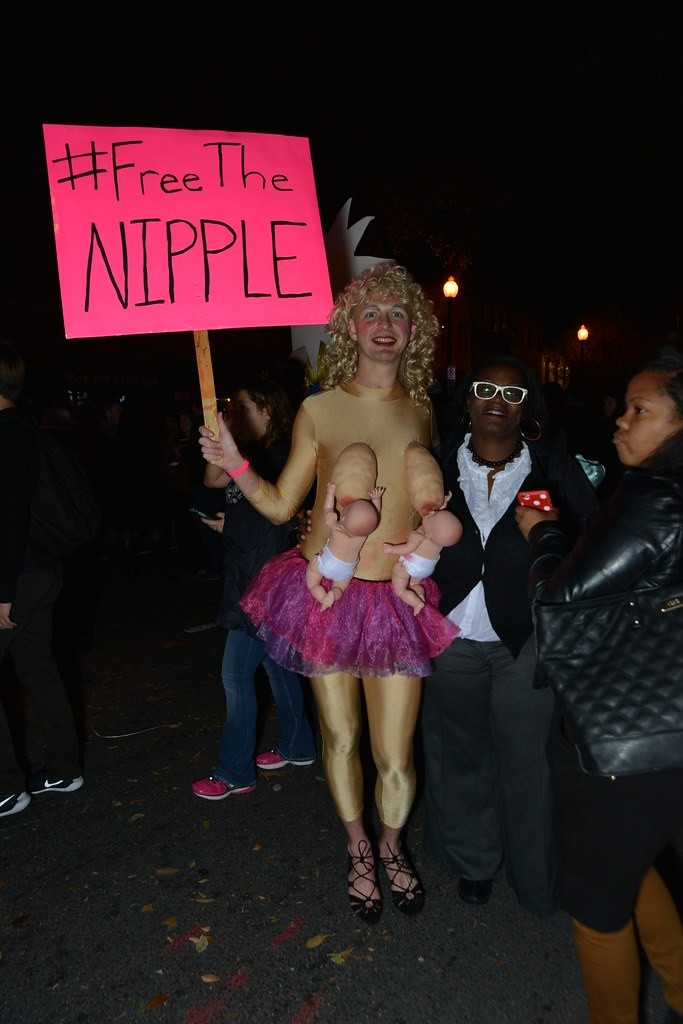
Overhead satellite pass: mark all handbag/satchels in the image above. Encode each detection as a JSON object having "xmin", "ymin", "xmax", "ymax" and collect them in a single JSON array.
[{"xmin": 534, "ymin": 584, "xmax": 683, "ymax": 790}]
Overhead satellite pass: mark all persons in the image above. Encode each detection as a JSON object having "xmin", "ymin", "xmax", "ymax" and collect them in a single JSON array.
[
  {"xmin": 186, "ymin": 373, "xmax": 317, "ymax": 799},
  {"xmin": 382, "ymin": 490, "xmax": 463, "ymax": 616},
  {"xmin": 199, "ymin": 255, "xmax": 446, "ymax": 922},
  {"xmin": 303, "ymin": 482, "xmax": 386, "ymax": 612},
  {"xmin": 294, "ymin": 350, "xmax": 602, "ymax": 920},
  {"xmin": 0, "ymin": 338, "xmax": 85, "ymax": 818},
  {"xmin": 510, "ymin": 349, "xmax": 683, "ymax": 1024},
  {"xmin": 66, "ymin": 384, "xmax": 208, "ymax": 558},
  {"xmin": 537, "ymin": 377, "xmax": 623, "ymax": 482}
]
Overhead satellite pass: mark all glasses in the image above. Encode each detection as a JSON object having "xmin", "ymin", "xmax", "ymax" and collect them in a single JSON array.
[{"xmin": 468, "ymin": 381, "xmax": 530, "ymax": 405}]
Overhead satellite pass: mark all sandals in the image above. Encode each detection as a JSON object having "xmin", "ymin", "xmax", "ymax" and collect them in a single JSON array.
[
  {"xmin": 377, "ymin": 841, "xmax": 425, "ymax": 916},
  {"xmin": 346, "ymin": 840, "xmax": 383, "ymax": 924}
]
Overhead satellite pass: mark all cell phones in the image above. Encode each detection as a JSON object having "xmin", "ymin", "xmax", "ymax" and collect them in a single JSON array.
[
  {"xmin": 189, "ymin": 507, "xmax": 214, "ymax": 520},
  {"xmin": 517, "ymin": 490, "xmax": 553, "ymax": 513}
]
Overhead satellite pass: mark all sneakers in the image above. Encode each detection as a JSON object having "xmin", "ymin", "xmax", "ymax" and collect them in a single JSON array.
[
  {"xmin": 192, "ymin": 775, "xmax": 256, "ymax": 800},
  {"xmin": 256, "ymin": 746, "xmax": 317, "ymax": 769},
  {"xmin": 27, "ymin": 771, "xmax": 85, "ymax": 794},
  {"xmin": 0, "ymin": 787, "xmax": 31, "ymax": 817}
]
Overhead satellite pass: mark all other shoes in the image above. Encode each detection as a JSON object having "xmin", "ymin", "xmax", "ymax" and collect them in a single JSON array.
[{"xmin": 459, "ymin": 875, "xmax": 491, "ymax": 905}]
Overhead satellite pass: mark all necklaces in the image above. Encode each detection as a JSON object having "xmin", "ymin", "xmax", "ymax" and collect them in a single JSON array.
[{"xmin": 467, "ymin": 435, "xmax": 523, "ymax": 468}]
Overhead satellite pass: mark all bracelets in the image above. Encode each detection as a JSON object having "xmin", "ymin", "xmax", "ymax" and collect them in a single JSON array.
[{"xmin": 228, "ymin": 458, "xmax": 249, "ymax": 476}]
